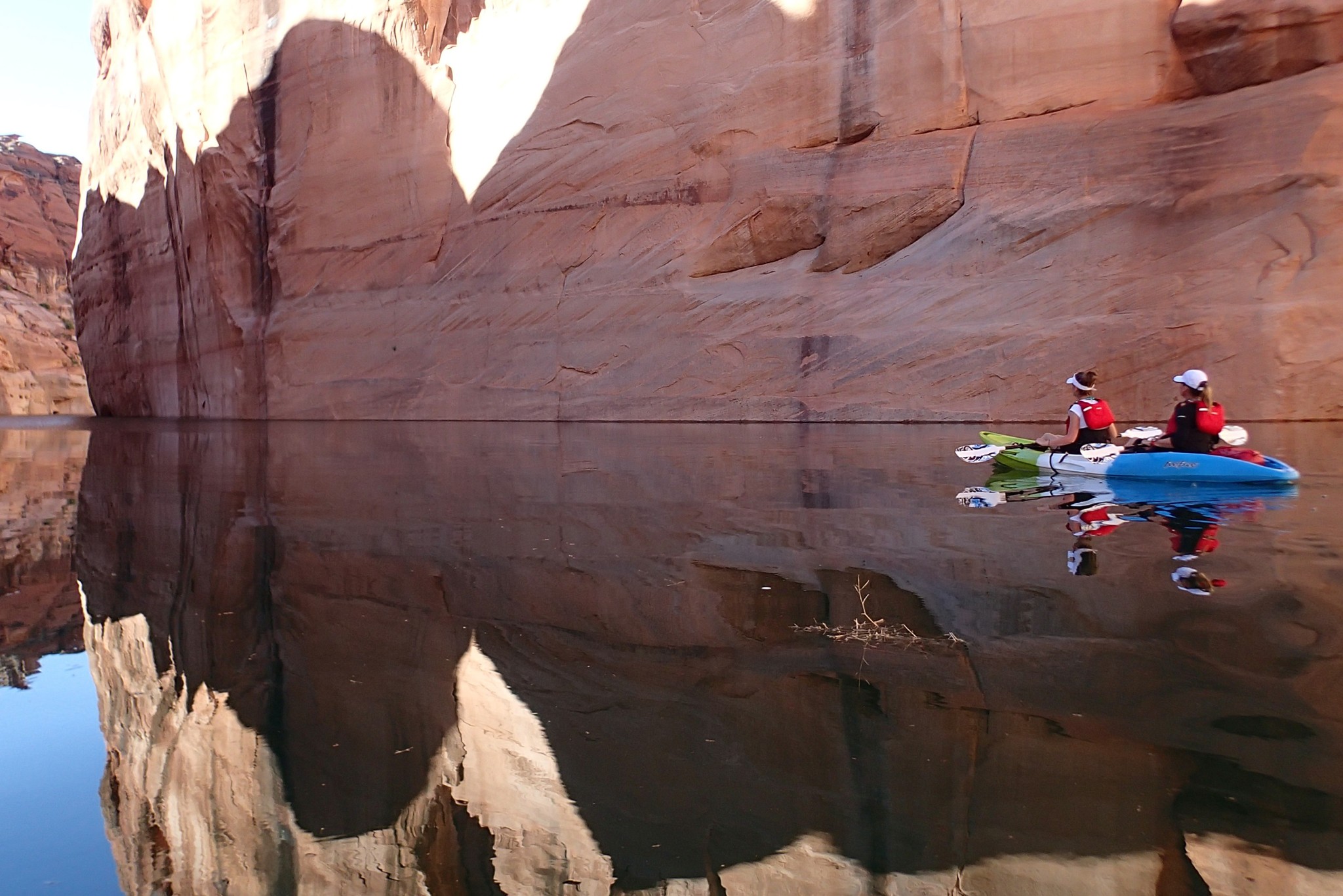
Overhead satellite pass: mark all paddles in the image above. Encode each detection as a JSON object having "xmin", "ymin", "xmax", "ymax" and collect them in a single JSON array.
[
  {"xmin": 1080, "ymin": 424, "xmax": 1248, "ymax": 465},
  {"xmin": 954, "ymin": 425, "xmax": 1163, "ymax": 465},
  {"xmin": 955, "ymin": 486, "xmax": 1023, "ymax": 508}
]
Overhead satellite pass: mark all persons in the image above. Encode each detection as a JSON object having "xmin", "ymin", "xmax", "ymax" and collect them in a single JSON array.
[
  {"xmin": 1036, "ymin": 493, "xmax": 1218, "ymax": 596},
  {"xmin": 1035, "ymin": 371, "xmax": 1117, "ymax": 454},
  {"xmin": 1125, "ymin": 369, "xmax": 1225, "ymax": 454}
]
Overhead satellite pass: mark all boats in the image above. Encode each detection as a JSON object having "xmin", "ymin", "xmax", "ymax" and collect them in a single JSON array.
[{"xmin": 978, "ymin": 430, "xmax": 1300, "ymax": 484}]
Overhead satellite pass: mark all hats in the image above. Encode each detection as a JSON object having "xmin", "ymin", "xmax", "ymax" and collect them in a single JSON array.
[
  {"xmin": 1172, "ymin": 369, "xmax": 1208, "ymax": 391},
  {"xmin": 1066, "ymin": 371, "xmax": 1097, "ymax": 391},
  {"xmin": 1067, "ymin": 548, "xmax": 1098, "ymax": 575},
  {"xmin": 1171, "ymin": 567, "xmax": 1198, "ymax": 582}
]
